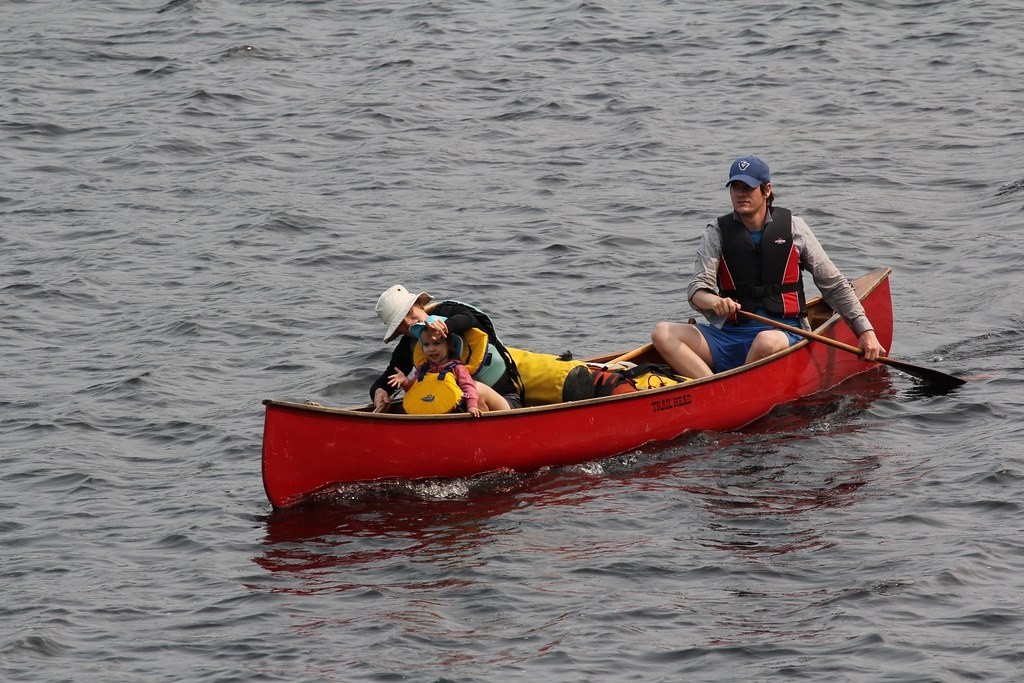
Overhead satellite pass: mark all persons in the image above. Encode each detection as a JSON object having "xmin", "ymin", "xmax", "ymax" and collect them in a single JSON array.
[
  {"xmin": 371, "ymin": 284, "xmax": 525, "ymax": 411},
  {"xmin": 387, "ymin": 315, "xmax": 483, "ymax": 417},
  {"xmin": 652, "ymin": 156, "xmax": 885, "ymax": 380}
]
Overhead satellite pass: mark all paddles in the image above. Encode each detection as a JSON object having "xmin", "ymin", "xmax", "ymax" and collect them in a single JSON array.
[{"xmin": 736, "ymin": 308, "xmax": 968, "ymax": 385}]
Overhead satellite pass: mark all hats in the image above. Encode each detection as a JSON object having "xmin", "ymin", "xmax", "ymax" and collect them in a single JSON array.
[
  {"xmin": 375, "ymin": 284, "xmax": 434, "ymax": 344},
  {"xmin": 409, "ymin": 315, "xmax": 463, "ymax": 361},
  {"xmin": 726, "ymin": 154, "xmax": 770, "ymax": 188}
]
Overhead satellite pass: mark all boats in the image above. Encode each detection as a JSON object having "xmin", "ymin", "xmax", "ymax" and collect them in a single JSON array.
[{"xmin": 262, "ymin": 267, "xmax": 894, "ymax": 508}]
